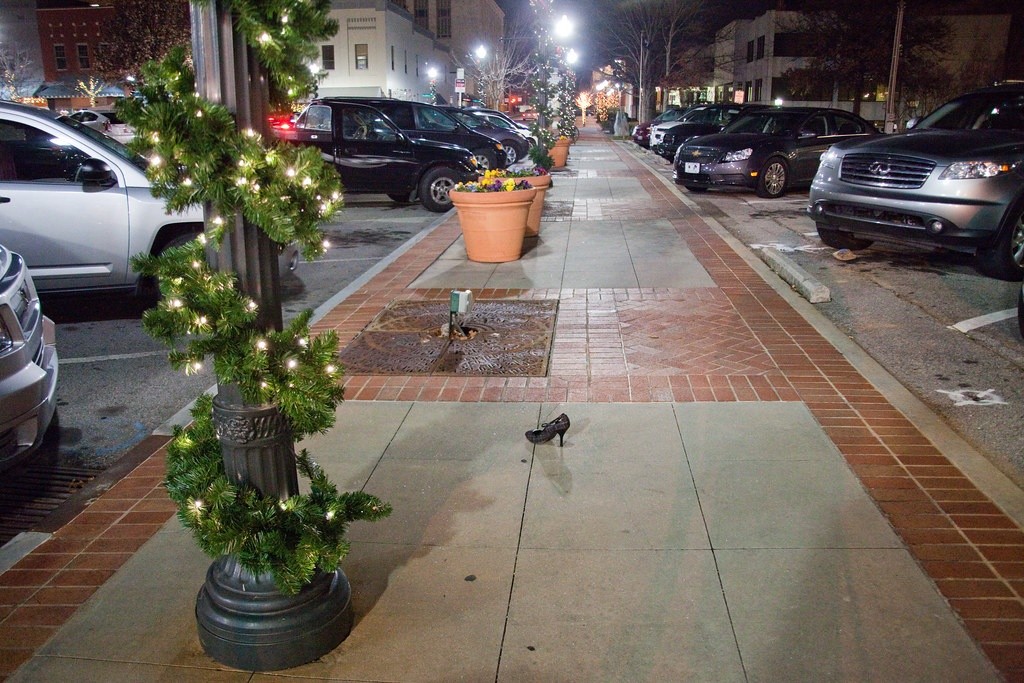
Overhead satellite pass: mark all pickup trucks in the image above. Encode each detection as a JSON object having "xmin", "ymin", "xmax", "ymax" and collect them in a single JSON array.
[{"xmin": 272, "ymin": 101, "xmax": 479, "ymax": 212}]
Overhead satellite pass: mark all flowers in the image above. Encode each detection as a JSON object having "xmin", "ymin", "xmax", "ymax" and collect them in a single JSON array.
[
  {"xmin": 454, "ymin": 168, "xmax": 548, "ymax": 192},
  {"xmin": 559, "ymin": 135, "xmax": 567, "ymax": 140}
]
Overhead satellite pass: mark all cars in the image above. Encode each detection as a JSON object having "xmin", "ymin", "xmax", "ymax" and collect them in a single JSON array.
[
  {"xmin": 0, "ymin": 101, "xmax": 205, "ymax": 473},
  {"xmin": 631, "ymin": 102, "xmax": 881, "ymax": 199}
]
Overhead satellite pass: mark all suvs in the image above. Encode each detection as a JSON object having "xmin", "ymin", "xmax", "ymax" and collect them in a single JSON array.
[
  {"xmin": 294, "ymin": 96, "xmax": 540, "ymax": 172},
  {"xmin": 805, "ymin": 80, "xmax": 1024, "ymax": 281}
]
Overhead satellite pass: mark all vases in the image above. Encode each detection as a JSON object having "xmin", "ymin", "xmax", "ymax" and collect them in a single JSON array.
[
  {"xmin": 449, "ymin": 189, "xmax": 537, "ymax": 263},
  {"xmin": 546, "ymin": 140, "xmax": 569, "ymax": 168},
  {"xmin": 478, "ymin": 173, "xmax": 551, "ymax": 237}
]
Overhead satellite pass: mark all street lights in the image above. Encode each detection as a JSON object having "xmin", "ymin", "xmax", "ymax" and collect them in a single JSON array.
[
  {"xmin": 554, "ymin": 15, "xmax": 571, "ymax": 115},
  {"xmin": 476, "ymin": 44, "xmax": 486, "ymax": 102}
]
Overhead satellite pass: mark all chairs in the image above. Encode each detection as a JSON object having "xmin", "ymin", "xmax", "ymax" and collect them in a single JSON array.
[
  {"xmin": 809, "ymin": 120, "xmax": 825, "ymax": 137},
  {"xmin": 838, "ymin": 124, "xmax": 857, "ymax": 135}
]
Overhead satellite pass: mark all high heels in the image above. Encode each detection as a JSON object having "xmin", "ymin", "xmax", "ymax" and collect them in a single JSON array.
[{"xmin": 525, "ymin": 413, "xmax": 570, "ymax": 447}]
